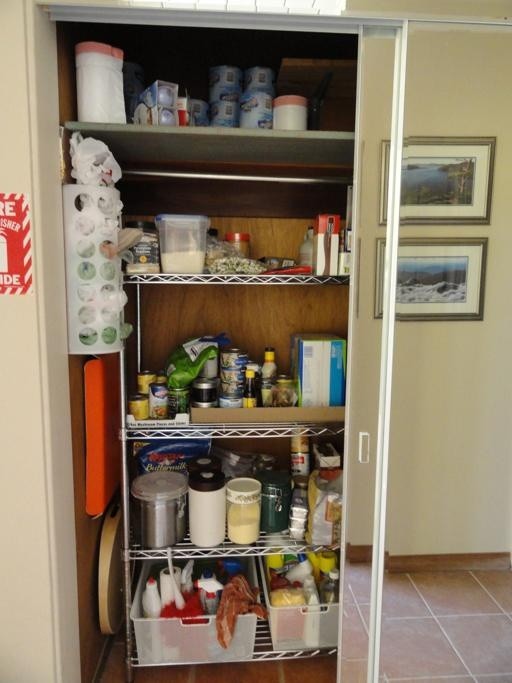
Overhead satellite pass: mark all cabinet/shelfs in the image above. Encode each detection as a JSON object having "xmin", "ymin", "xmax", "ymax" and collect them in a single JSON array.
[{"xmin": 39, "ymin": 4, "xmax": 362, "ymax": 683}]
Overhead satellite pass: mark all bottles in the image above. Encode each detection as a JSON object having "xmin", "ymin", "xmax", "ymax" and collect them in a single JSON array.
[
  {"xmin": 127, "ymin": 394, "xmax": 149, "ymax": 421},
  {"xmin": 246, "ymin": 364, "xmax": 263, "ymax": 408},
  {"xmin": 227, "ymin": 477, "xmax": 262, "ymax": 545},
  {"xmin": 188, "ymin": 469, "xmax": 228, "ymax": 549},
  {"xmin": 293, "ymin": 476, "xmax": 309, "ymax": 508},
  {"xmin": 319, "ymin": 568, "xmax": 340, "ymax": 610},
  {"xmin": 193, "ymin": 378, "xmax": 218, "ymax": 401},
  {"xmin": 168, "ymin": 386, "xmax": 191, "ymax": 420},
  {"xmin": 147, "ymin": 382, "xmax": 168, "ymax": 420},
  {"xmin": 125, "ymin": 220, "xmax": 161, "ymax": 276},
  {"xmin": 224, "ymin": 231, "xmax": 252, "ymax": 260},
  {"xmin": 272, "ymin": 375, "xmax": 297, "ymax": 408},
  {"xmin": 298, "ymin": 225, "xmax": 316, "ymax": 269},
  {"xmin": 260, "ymin": 346, "xmax": 277, "ymax": 408},
  {"xmin": 259, "ymin": 468, "xmax": 294, "ymax": 534},
  {"xmin": 137, "ymin": 370, "xmax": 157, "ymax": 395},
  {"xmin": 130, "ymin": 471, "xmax": 188, "ymax": 548},
  {"xmin": 242, "ymin": 369, "xmax": 258, "ymax": 409}
]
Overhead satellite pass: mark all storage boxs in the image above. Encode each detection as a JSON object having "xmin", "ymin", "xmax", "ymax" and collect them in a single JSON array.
[
  {"xmin": 130, "ymin": 564, "xmax": 261, "ymax": 666},
  {"xmin": 258, "ymin": 556, "xmax": 339, "ymax": 652}
]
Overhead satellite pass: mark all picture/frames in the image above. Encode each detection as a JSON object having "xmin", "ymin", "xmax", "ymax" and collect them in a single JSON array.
[
  {"xmin": 374, "ymin": 238, "xmax": 488, "ymax": 321},
  {"xmin": 378, "ymin": 137, "xmax": 496, "ymax": 225}
]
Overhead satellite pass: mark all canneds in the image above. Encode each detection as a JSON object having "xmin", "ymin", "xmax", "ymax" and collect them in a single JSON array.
[
  {"xmin": 219, "ymin": 384, "xmax": 246, "ymax": 399},
  {"xmin": 220, "ymin": 369, "xmax": 247, "ymax": 384},
  {"xmin": 219, "ymin": 348, "xmax": 248, "ymax": 369}
]
